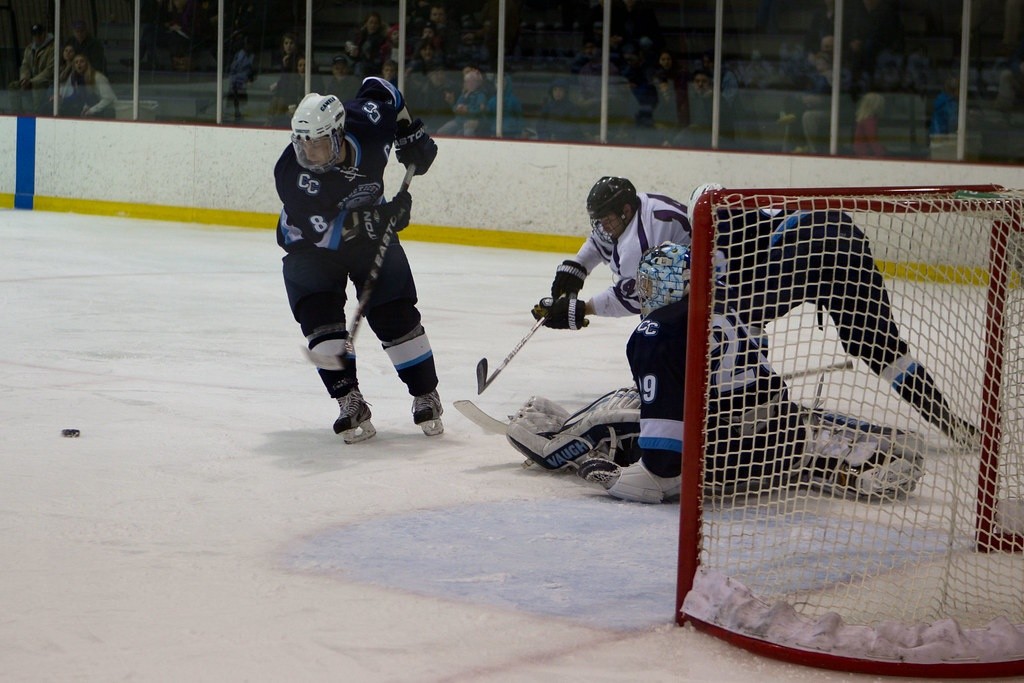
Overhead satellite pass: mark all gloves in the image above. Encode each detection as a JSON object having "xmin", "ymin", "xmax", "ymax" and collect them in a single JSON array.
[
  {"xmin": 531, "ymin": 297, "xmax": 589, "ymax": 330},
  {"xmin": 359, "ymin": 192, "xmax": 412, "ymax": 242},
  {"xmin": 394, "ymin": 118, "xmax": 438, "ymax": 176},
  {"xmin": 551, "ymin": 259, "xmax": 587, "ymax": 299}
]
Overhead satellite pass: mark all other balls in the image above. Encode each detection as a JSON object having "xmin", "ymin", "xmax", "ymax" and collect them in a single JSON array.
[{"xmin": 62, "ymin": 428, "xmax": 80, "ymax": 438}]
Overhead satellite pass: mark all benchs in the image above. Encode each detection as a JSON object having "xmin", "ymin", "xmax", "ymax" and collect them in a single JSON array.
[{"xmin": 0, "ymin": 0, "xmax": 1024, "ymax": 164}]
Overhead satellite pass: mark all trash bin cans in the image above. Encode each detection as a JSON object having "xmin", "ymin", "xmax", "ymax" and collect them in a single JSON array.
[
  {"xmin": 115, "ymin": 99, "xmax": 160, "ymax": 121},
  {"xmin": 929, "ymin": 134, "xmax": 986, "ymax": 161}
]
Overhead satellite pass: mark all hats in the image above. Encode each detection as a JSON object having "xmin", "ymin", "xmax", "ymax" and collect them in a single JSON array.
[
  {"xmin": 72, "ymin": 20, "xmax": 87, "ymax": 30},
  {"xmin": 31, "ymin": 23, "xmax": 43, "ymax": 37}
]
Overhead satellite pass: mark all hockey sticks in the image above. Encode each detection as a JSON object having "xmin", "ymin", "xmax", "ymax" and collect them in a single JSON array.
[
  {"xmin": 476, "ymin": 292, "xmax": 568, "ymax": 397},
  {"xmin": 453, "ymin": 359, "xmax": 856, "ymax": 441},
  {"xmin": 299, "ymin": 162, "xmax": 416, "ymax": 371}
]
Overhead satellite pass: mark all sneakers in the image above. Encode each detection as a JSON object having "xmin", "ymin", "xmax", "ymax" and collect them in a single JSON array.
[
  {"xmin": 410, "ymin": 389, "xmax": 445, "ymax": 437},
  {"xmin": 934, "ymin": 412, "xmax": 982, "ymax": 449},
  {"xmin": 332, "ymin": 386, "xmax": 377, "ymax": 445}
]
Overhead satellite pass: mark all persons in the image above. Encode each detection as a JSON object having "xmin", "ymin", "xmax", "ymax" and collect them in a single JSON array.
[
  {"xmin": 274, "ymin": 76, "xmax": 443, "ymax": 435},
  {"xmin": 505, "ymin": 240, "xmax": 925, "ymax": 505},
  {"xmin": 120, "ymin": 0, "xmax": 258, "ymax": 71},
  {"xmin": 230, "ymin": 34, "xmax": 256, "ymax": 86},
  {"xmin": 688, "ymin": 183, "xmax": 978, "ymax": 443},
  {"xmin": 266, "ymin": 33, "xmax": 325, "ymax": 126},
  {"xmin": 325, "ymin": 0, "xmax": 524, "ymax": 134},
  {"xmin": 7, "ymin": 19, "xmax": 117, "ymax": 118},
  {"xmin": 520, "ymin": 0, "xmax": 735, "ymax": 150},
  {"xmin": 530, "ymin": 176, "xmax": 728, "ymax": 330},
  {"xmin": 768, "ymin": 0, "xmax": 1024, "ymax": 161}
]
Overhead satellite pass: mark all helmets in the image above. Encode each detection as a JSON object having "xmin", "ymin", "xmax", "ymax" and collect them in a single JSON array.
[
  {"xmin": 586, "ymin": 176, "xmax": 638, "ymax": 242},
  {"xmin": 634, "ymin": 242, "xmax": 691, "ymax": 318},
  {"xmin": 290, "ymin": 92, "xmax": 346, "ymax": 175}
]
